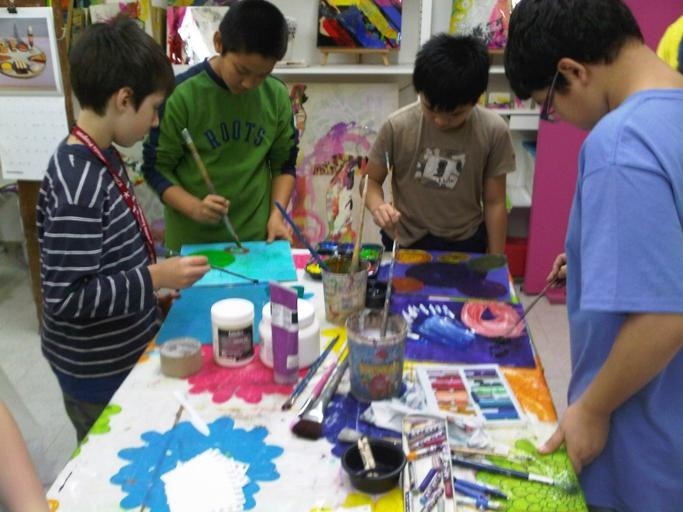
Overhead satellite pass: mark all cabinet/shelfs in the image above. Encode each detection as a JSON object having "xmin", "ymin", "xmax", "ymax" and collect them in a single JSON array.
[{"xmin": 489, "ymin": 63, "xmax": 541, "ymax": 212}]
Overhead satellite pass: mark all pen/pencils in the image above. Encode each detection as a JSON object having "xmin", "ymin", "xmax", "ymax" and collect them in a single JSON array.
[
  {"xmin": 336, "ymin": 252, "xmax": 342, "ymax": 273},
  {"xmin": 406, "ymin": 369, "xmax": 520, "ymax": 512}
]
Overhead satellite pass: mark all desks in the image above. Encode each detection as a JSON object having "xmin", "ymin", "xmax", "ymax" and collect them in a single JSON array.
[{"xmin": 43, "ymin": 248, "xmax": 589, "ymax": 509}]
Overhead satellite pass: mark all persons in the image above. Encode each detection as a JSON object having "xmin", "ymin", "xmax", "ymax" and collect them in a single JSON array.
[
  {"xmin": 0, "ymin": 402, "xmax": 47, "ymax": 509},
  {"xmin": 138, "ymin": 1, "xmax": 301, "ymax": 254},
  {"xmin": 360, "ymin": 32, "xmax": 517, "ymax": 254},
  {"xmin": 33, "ymin": 15, "xmax": 210, "ymax": 443},
  {"xmin": 656, "ymin": 11, "xmax": 683, "ymax": 73},
  {"xmin": 502, "ymin": 0, "xmax": 683, "ymax": 512}
]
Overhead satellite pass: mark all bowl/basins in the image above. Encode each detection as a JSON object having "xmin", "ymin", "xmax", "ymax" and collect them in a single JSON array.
[{"xmin": 341, "ymin": 440, "xmax": 406, "ymax": 493}]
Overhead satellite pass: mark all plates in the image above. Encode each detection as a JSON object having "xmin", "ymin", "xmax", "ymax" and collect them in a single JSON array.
[{"xmin": 0, "ymin": 40, "xmax": 45, "ymax": 78}]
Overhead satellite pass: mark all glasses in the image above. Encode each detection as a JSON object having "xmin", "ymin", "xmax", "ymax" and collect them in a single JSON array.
[{"xmin": 538, "ymin": 68, "xmax": 562, "ymax": 125}]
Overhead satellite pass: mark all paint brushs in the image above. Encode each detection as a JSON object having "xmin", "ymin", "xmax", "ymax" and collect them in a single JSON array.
[
  {"xmin": 165, "ymin": 255, "xmax": 258, "ymax": 284},
  {"xmin": 180, "ymin": 128, "xmax": 242, "ymax": 248},
  {"xmin": 337, "ymin": 428, "xmax": 554, "ymax": 486},
  {"xmin": 274, "ymin": 201, "xmax": 328, "ymax": 271},
  {"xmin": 385, "ymin": 153, "xmax": 399, "ymax": 260},
  {"xmin": 380, "ymin": 229, "xmax": 401, "ymax": 337},
  {"xmin": 140, "ymin": 406, "xmax": 184, "ymax": 512},
  {"xmin": 350, "ymin": 174, "xmax": 368, "ymax": 274},
  {"xmin": 499, "ymin": 276, "xmax": 558, "ymax": 342},
  {"xmin": 281, "ymin": 335, "xmax": 349, "ymax": 439}
]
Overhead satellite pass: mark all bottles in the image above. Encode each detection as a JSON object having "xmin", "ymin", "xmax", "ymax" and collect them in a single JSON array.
[
  {"xmin": 210, "ymin": 295, "xmax": 254, "ymax": 368},
  {"xmin": 258, "ymin": 299, "xmax": 321, "ymax": 369}
]
